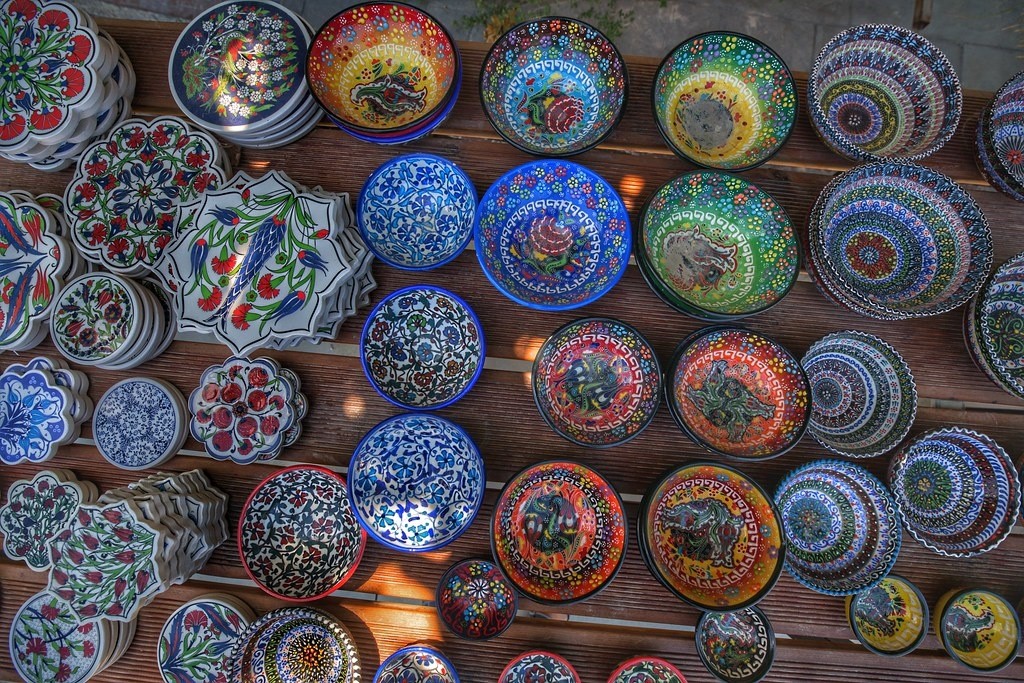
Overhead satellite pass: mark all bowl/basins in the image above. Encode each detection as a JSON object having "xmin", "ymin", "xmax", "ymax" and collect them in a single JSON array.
[{"xmin": 217, "ymin": 1, "xmax": 1023, "ymax": 682}]
